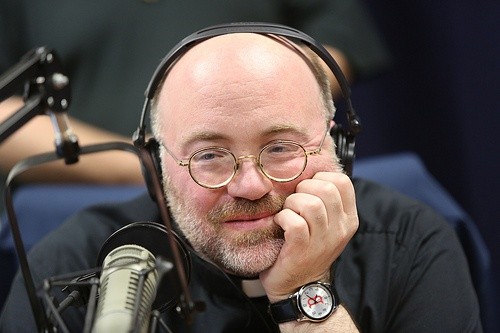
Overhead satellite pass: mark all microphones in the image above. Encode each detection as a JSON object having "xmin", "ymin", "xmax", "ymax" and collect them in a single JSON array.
[{"xmin": 91, "ymin": 244, "xmax": 160, "ymax": 333}]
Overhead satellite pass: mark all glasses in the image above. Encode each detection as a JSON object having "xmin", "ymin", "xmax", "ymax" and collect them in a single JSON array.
[{"xmin": 160, "ymin": 124, "xmax": 329, "ymax": 190}]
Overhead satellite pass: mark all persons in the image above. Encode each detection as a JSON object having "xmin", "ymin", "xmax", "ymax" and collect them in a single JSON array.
[
  {"xmin": 0, "ymin": 0, "xmax": 381, "ymax": 189},
  {"xmin": 0, "ymin": 23, "xmax": 486, "ymax": 333}
]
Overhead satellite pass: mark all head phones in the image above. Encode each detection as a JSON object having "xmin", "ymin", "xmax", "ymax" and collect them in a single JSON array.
[{"xmin": 133, "ymin": 22, "xmax": 359, "ymax": 208}]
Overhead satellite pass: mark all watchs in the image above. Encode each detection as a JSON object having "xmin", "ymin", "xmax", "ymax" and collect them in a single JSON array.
[{"xmin": 270, "ymin": 281, "xmax": 339, "ymax": 324}]
